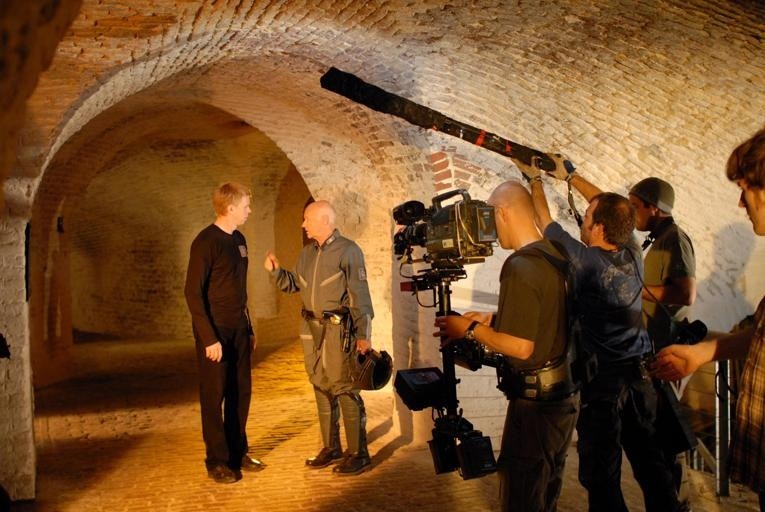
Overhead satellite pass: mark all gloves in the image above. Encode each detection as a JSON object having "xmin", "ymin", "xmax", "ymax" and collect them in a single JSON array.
[
  {"xmin": 511, "ymin": 155, "xmax": 542, "ymax": 184},
  {"xmin": 546, "ymin": 153, "xmax": 577, "ymax": 182}
]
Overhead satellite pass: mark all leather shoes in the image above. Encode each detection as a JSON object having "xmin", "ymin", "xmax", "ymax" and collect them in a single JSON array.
[
  {"xmin": 209, "ymin": 466, "xmax": 236, "ymax": 483},
  {"xmin": 306, "ymin": 448, "xmax": 343, "ymax": 468},
  {"xmin": 333, "ymin": 456, "xmax": 371, "ymax": 476},
  {"xmin": 241, "ymin": 453, "xmax": 262, "ymax": 471}
]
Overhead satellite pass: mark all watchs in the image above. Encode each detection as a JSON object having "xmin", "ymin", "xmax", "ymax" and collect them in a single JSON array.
[{"xmin": 464, "ymin": 322, "xmax": 479, "ymax": 341}]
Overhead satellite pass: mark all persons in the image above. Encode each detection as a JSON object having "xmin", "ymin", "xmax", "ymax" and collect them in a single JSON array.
[
  {"xmin": 651, "ymin": 128, "xmax": 765, "ymax": 512},
  {"xmin": 265, "ymin": 200, "xmax": 373, "ymax": 476},
  {"xmin": 433, "ymin": 180, "xmax": 580, "ymax": 512},
  {"xmin": 513, "ymin": 152, "xmax": 692, "ymax": 512},
  {"xmin": 628, "ymin": 178, "xmax": 699, "ymax": 373},
  {"xmin": 184, "ymin": 181, "xmax": 266, "ymax": 482}
]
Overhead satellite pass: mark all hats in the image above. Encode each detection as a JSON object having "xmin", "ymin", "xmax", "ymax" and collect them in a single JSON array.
[{"xmin": 630, "ymin": 177, "xmax": 674, "ymax": 212}]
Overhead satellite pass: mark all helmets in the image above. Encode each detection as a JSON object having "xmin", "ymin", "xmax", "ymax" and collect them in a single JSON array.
[{"xmin": 350, "ymin": 349, "xmax": 392, "ymax": 390}]
